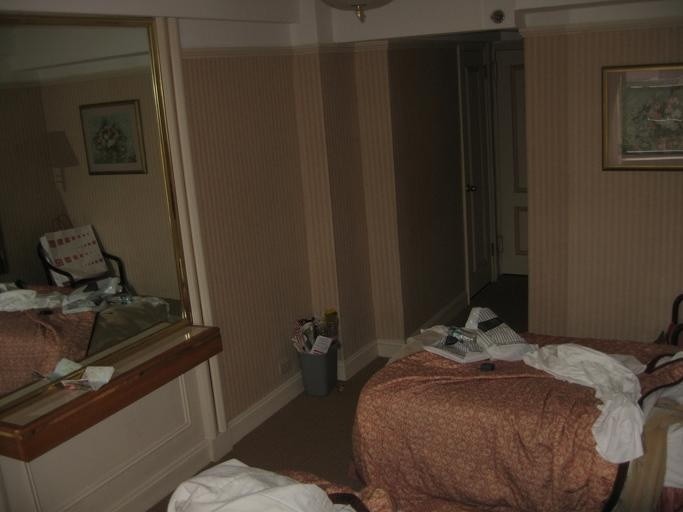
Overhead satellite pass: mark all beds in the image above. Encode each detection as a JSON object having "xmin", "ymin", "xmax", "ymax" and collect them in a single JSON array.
[
  {"xmin": 167, "ymin": 456, "xmax": 399, "ymax": 512},
  {"xmin": 351, "ymin": 325, "xmax": 683, "ymax": 512},
  {"xmin": 0, "ymin": 280, "xmax": 169, "ymax": 398}
]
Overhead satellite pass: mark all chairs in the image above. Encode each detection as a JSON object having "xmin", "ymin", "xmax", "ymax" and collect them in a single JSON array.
[
  {"xmin": 35, "ymin": 239, "xmax": 127, "ymax": 294},
  {"xmin": 667, "ymin": 294, "xmax": 683, "ymax": 346}
]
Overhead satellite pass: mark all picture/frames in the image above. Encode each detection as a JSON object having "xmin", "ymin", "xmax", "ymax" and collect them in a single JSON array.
[
  {"xmin": 78, "ymin": 98, "xmax": 148, "ymax": 176},
  {"xmin": 598, "ymin": 61, "xmax": 683, "ymax": 171}
]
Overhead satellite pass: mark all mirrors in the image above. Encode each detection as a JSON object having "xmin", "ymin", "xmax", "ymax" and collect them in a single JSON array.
[{"xmin": 0, "ymin": 6, "xmax": 190, "ymax": 415}]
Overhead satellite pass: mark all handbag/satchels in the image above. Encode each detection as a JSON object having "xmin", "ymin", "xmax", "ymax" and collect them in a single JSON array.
[{"xmin": 40, "ymin": 214, "xmax": 108, "ymax": 287}]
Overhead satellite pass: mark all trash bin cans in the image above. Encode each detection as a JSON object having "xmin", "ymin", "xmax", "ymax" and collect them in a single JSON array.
[{"xmin": 299, "ymin": 341, "xmax": 341, "ymax": 396}]
